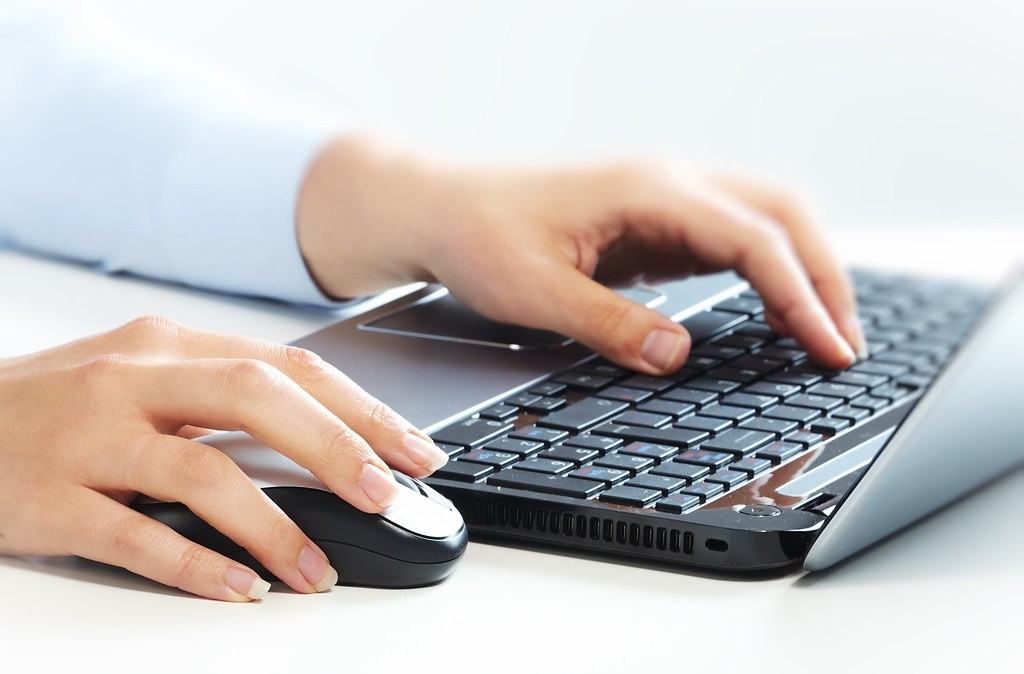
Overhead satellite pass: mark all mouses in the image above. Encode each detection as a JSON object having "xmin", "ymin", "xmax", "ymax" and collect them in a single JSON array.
[{"xmin": 117, "ymin": 432, "xmax": 468, "ymax": 588}]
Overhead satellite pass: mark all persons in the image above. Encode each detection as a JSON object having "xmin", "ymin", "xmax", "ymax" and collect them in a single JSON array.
[{"xmin": 0, "ymin": 0, "xmax": 870, "ymax": 603}]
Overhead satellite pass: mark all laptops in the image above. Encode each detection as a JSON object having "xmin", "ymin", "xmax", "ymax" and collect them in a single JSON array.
[{"xmin": 209, "ymin": 255, "xmax": 1024, "ymax": 583}]
